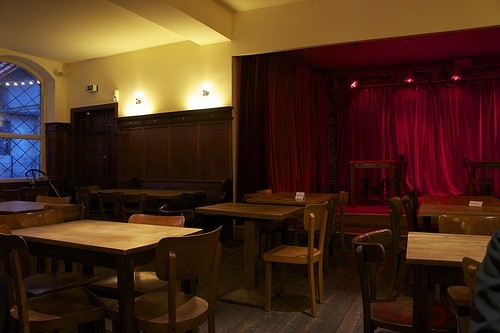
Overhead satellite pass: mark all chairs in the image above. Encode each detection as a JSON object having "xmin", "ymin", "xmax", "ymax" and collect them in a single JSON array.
[{"xmin": 0, "ymin": 155, "xmax": 500, "ymax": 333}]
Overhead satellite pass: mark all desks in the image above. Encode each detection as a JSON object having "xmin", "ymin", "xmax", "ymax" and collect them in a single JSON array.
[
  {"xmin": 12, "ymin": 219, "xmax": 203, "ymax": 333},
  {"xmin": 349, "ymin": 160, "xmax": 403, "ymax": 208},
  {"xmin": 0, "ymin": 201, "xmax": 76, "ymax": 214},
  {"xmin": 246, "ymin": 191, "xmax": 339, "ymax": 245},
  {"xmin": 195, "ymin": 203, "xmax": 305, "ymax": 307},
  {"xmin": 406, "ymin": 232, "xmax": 493, "ymax": 306},
  {"xmin": 419, "ymin": 196, "xmax": 500, "ymax": 236},
  {"xmin": 471, "ymin": 161, "xmax": 500, "ymax": 195},
  {"xmin": 89, "ymin": 189, "xmax": 202, "ymax": 214}
]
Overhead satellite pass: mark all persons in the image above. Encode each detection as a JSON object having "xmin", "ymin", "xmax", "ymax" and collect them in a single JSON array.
[{"xmin": 466, "ymin": 227, "xmax": 500, "ymax": 332}]
